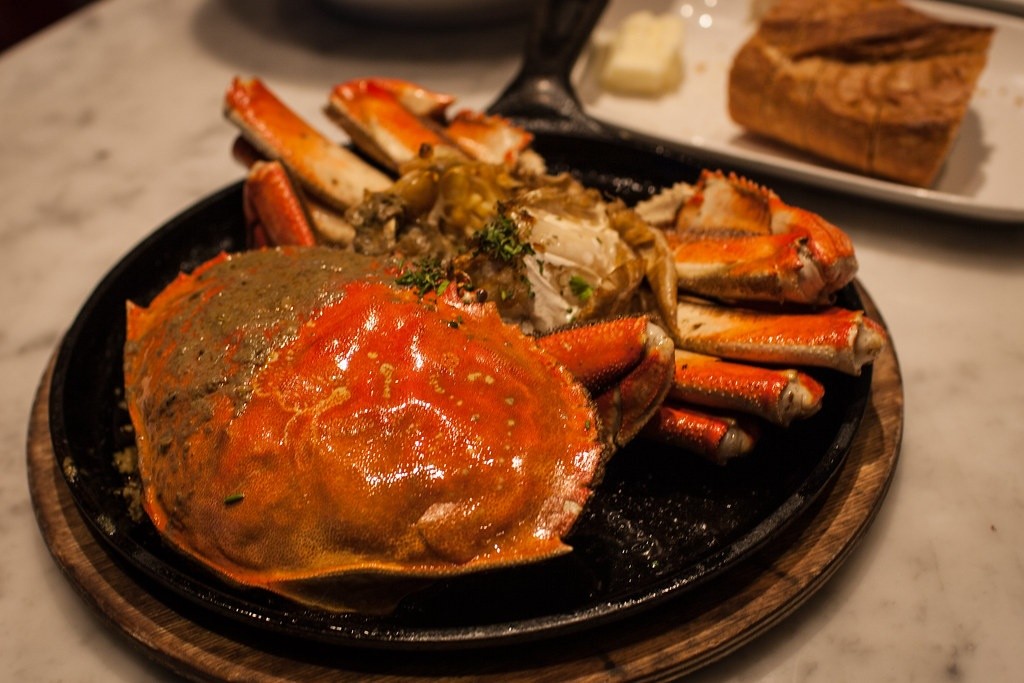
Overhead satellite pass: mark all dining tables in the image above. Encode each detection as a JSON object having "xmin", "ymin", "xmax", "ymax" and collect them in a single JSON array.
[{"xmin": 0, "ymin": 2, "xmax": 1023, "ymax": 681}]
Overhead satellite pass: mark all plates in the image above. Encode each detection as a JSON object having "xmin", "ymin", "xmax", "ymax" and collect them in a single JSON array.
[
  {"xmin": 25, "ymin": 277, "xmax": 903, "ymax": 683},
  {"xmin": 572, "ymin": 1, "xmax": 1020, "ymax": 224}
]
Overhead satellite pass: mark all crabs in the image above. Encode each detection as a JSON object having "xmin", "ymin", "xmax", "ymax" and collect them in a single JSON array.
[
  {"xmin": 121, "ymin": 160, "xmax": 678, "ymax": 617},
  {"xmin": 221, "ymin": 74, "xmax": 889, "ymax": 468}
]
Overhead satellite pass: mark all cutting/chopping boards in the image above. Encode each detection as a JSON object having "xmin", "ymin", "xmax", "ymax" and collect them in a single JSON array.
[{"xmin": 48, "ymin": 0, "xmax": 873, "ymax": 657}]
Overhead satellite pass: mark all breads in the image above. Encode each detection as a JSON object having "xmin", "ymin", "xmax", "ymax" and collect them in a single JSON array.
[{"xmin": 725, "ymin": 0, "xmax": 998, "ymax": 189}]
